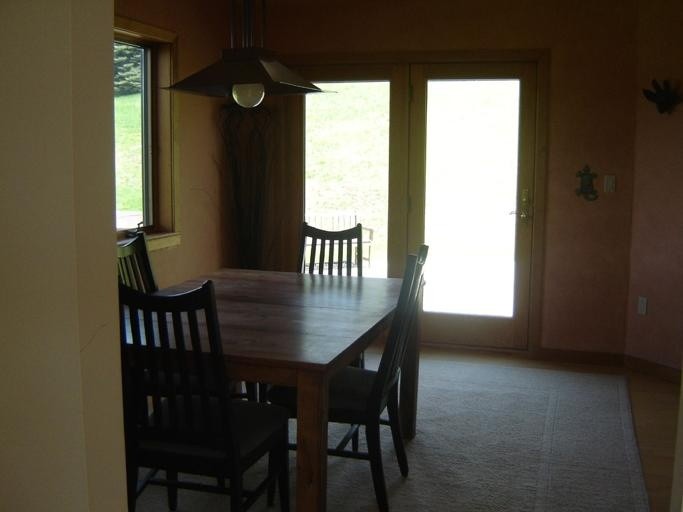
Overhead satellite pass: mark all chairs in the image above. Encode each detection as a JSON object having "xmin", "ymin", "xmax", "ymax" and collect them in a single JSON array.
[
  {"xmin": 266, "ymin": 243, "xmax": 429, "ymax": 512},
  {"xmin": 117, "ymin": 232, "xmax": 156, "ymax": 293},
  {"xmin": 120, "ymin": 280, "xmax": 290, "ymax": 511}
]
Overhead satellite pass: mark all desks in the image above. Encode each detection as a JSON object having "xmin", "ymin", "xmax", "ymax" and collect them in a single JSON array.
[{"xmin": 121, "ymin": 268, "xmax": 426, "ymax": 512}]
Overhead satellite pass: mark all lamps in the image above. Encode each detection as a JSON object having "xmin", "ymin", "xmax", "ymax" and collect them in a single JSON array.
[{"xmin": 157, "ymin": 1, "xmax": 336, "ymax": 109}]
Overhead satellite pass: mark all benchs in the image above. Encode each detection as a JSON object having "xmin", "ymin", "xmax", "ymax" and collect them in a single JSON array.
[{"xmin": 297, "ymin": 222, "xmax": 366, "ymax": 369}]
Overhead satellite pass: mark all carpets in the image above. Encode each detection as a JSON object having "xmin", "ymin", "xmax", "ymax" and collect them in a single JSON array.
[{"xmin": 131, "ymin": 352, "xmax": 649, "ymax": 512}]
